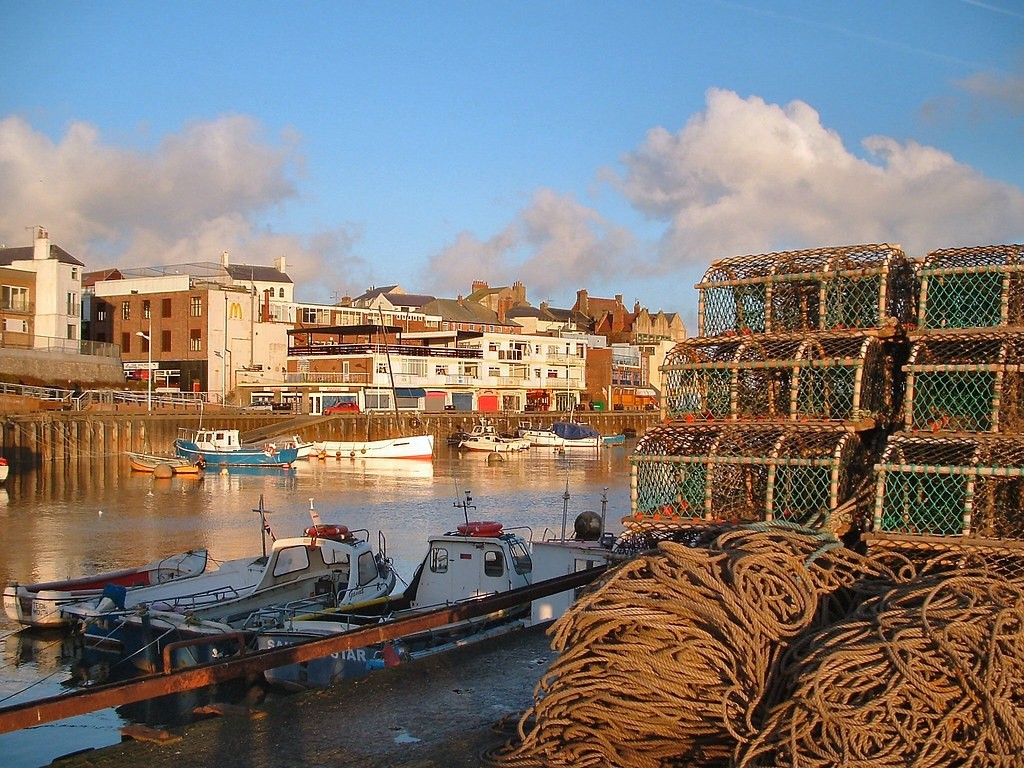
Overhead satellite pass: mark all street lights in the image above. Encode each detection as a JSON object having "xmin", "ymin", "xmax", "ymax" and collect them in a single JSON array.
[{"xmin": 136, "ymin": 331, "xmax": 151, "ymax": 417}]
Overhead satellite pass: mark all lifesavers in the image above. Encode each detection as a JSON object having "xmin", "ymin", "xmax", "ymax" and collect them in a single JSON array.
[
  {"xmin": 457, "ymin": 522, "xmax": 503, "ymax": 537},
  {"xmin": 264, "ymin": 444, "xmax": 276, "ymax": 458},
  {"xmin": 305, "ymin": 523, "xmax": 354, "ymax": 541},
  {"xmin": 409, "ymin": 417, "xmax": 421, "ymax": 429}
]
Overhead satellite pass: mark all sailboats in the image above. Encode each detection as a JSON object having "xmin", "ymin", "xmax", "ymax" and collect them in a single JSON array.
[
  {"xmin": 517, "ymin": 348, "xmax": 626, "ymax": 446},
  {"xmin": 293, "ymin": 305, "xmax": 435, "ymax": 459}
]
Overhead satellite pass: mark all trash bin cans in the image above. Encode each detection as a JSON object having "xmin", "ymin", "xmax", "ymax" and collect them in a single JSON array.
[
  {"xmin": 445, "ymin": 405, "xmax": 455, "ymax": 414},
  {"xmin": 576, "ymin": 404, "xmax": 585, "ymax": 412},
  {"xmin": 272, "ymin": 403, "xmax": 291, "ymax": 415},
  {"xmin": 645, "ymin": 404, "xmax": 654, "ymax": 412},
  {"xmin": 614, "ymin": 404, "xmax": 624, "ymax": 413},
  {"xmin": 524, "ymin": 405, "xmax": 535, "ymax": 414}
]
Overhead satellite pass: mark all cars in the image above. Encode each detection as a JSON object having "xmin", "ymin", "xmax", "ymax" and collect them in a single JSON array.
[
  {"xmin": 322, "ymin": 401, "xmax": 360, "ymax": 416},
  {"xmin": 241, "ymin": 401, "xmax": 272, "ymax": 411},
  {"xmin": 272, "ymin": 402, "xmax": 292, "ymax": 415}
]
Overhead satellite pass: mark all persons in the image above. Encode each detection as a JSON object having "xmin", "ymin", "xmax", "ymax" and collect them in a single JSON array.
[{"xmin": 589, "ymin": 399, "xmax": 594, "ymax": 410}]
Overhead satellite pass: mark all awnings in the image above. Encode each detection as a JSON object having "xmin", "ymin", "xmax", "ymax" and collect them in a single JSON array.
[
  {"xmin": 132, "ymin": 370, "xmax": 155, "ymax": 380},
  {"xmin": 635, "ymin": 388, "xmax": 656, "ymax": 396},
  {"xmin": 394, "ymin": 388, "xmax": 427, "ymax": 398}
]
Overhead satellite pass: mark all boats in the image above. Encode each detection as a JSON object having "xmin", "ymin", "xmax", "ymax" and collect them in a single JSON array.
[
  {"xmin": 2, "ymin": 546, "xmax": 209, "ymax": 628},
  {"xmin": 446, "ymin": 424, "xmax": 500, "ymax": 446},
  {"xmin": 296, "ymin": 445, "xmax": 312, "ymax": 456},
  {"xmin": 124, "ymin": 451, "xmax": 198, "ymax": 473},
  {"xmin": 57, "ymin": 446, "xmax": 535, "ymax": 704},
  {"xmin": 174, "ymin": 427, "xmax": 300, "ymax": 467},
  {"xmin": 54, "ymin": 498, "xmax": 397, "ymax": 675},
  {"xmin": 524, "ymin": 421, "xmax": 603, "ymax": 447},
  {"xmin": 460, "ymin": 435, "xmax": 532, "ymax": 452}
]
